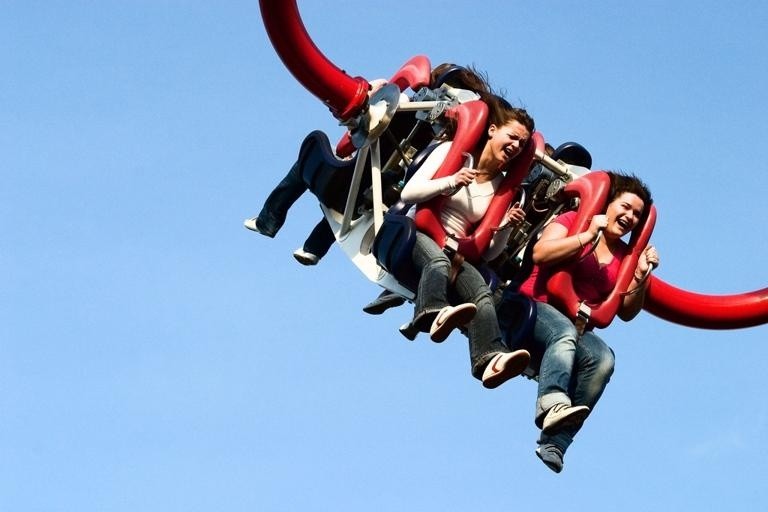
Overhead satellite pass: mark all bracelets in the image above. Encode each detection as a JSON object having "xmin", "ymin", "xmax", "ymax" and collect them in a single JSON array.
[
  {"xmin": 631, "ymin": 269, "xmax": 643, "ymax": 282},
  {"xmin": 577, "ymin": 233, "xmax": 583, "ymax": 249}
]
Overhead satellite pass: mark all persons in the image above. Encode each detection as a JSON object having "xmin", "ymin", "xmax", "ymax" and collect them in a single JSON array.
[
  {"xmin": 241, "ymin": 60, "xmax": 479, "ymax": 264},
  {"xmin": 410, "ymin": 108, "xmax": 536, "ymax": 387},
  {"xmin": 364, "ymin": 285, "xmax": 417, "ymax": 340},
  {"xmin": 524, "ymin": 178, "xmax": 659, "ymax": 473}
]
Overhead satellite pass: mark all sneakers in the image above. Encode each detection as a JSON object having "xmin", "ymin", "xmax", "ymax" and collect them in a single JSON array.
[
  {"xmin": 542, "ymin": 402, "xmax": 592, "ymax": 434},
  {"xmin": 533, "ymin": 442, "xmax": 564, "ymax": 474},
  {"xmin": 429, "ymin": 303, "xmax": 478, "ymax": 343},
  {"xmin": 398, "ymin": 320, "xmax": 419, "ymax": 341},
  {"xmin": 244, "ymin": 218, "xmax": 261, "ymax": 234},
  {"xmin": 362, "ymin": 290, "xmax": 406, "ymax": 315},
  {"xmin": 480, "ymin": 348, "xmax": 532, "ymax": 390},
  {"xmin": 291, "ymin": 247, "xmax": 320, "ymax": 266}
]
